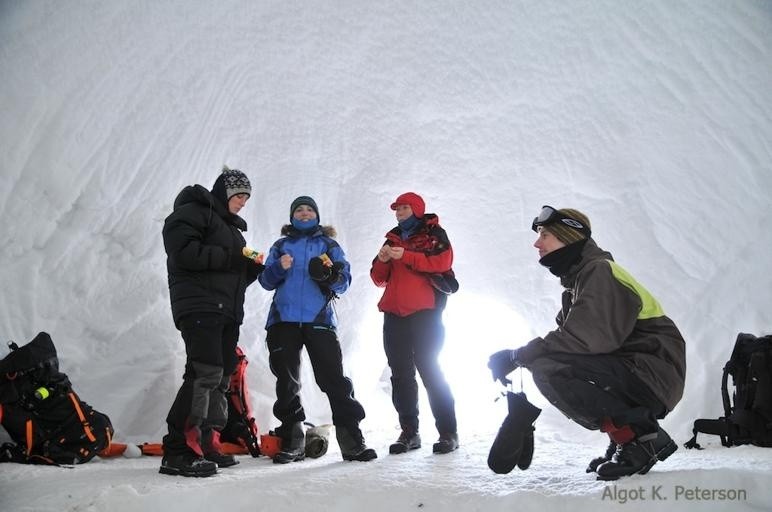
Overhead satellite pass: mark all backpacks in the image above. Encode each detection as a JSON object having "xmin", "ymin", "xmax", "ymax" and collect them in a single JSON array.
[
  {"xmin": 186, "ymin": 345, "xmax": 258, "ymax": 454},
  {"xmin": 0, "ymin": 330, "xmax": 116, "ymax": 467},
  {"xmin": 683, "ymin": 332, "xmax": 772, "ymax": 450}
]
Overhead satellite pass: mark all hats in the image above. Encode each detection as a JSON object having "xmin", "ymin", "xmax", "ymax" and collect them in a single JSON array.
[
  {"xmin": 289, "ymin": 196, "xmax": 323, "ymax": 223},
  {"xmin": 531, "ymin": 205, "xmax": 592, "ymax": 245},
  {"xmin": 389, "ymin": 192, "xmax": 426, "ymax": 219},
  {"xmin": 218, "ymin": 167, "xmax": 253, "ymax": 198}
]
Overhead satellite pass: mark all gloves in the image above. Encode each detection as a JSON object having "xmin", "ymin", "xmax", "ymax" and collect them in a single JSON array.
[
  {"xmin": 309, "ymin": 257, "xmax": 339, "ymax": 286},
  {"xmin": 488, "ymin": 389, "xmax": 541, "ymax": 474}
]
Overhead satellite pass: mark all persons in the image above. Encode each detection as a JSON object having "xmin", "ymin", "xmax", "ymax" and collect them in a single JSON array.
[
  {"xmin": 159, "ymin": 169, "xmax": 266, "ymax": 477},
  {"xmin": 371, "ymin": 192, "xmax": 458, "ymax": 453},
  {"xmin": 488, "ymin": 206, "xmax": 687, "ymax": 481},
  {"xmin": 259, "ymin": 197, "xmax": 377, "ymax": 463}
]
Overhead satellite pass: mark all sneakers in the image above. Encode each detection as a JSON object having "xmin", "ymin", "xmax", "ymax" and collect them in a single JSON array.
[
  {"xmin": 207, "ymin": 452, "xmax": 240, "ymax": 468},
  {"xmin": 342, "ymin": 447, "xmax": 377, "ymax": 462},
  {"xmin": 432, "ymin": 432, "xmax": 459, "ymax": 454},
  {"xmin": 388, "ymin": 429, "xmax": 422, "ymax": 455},
  {"xmin": 161, "ymin": 451, "xmax": 218, "ymax": 476},
  {"xmin": 588, "ymin": 426, "xmax": 680, "ymax": 481},
  {"xmin": 272, "ymin": 448, "xmax": 305, "ymax": 465}
]
